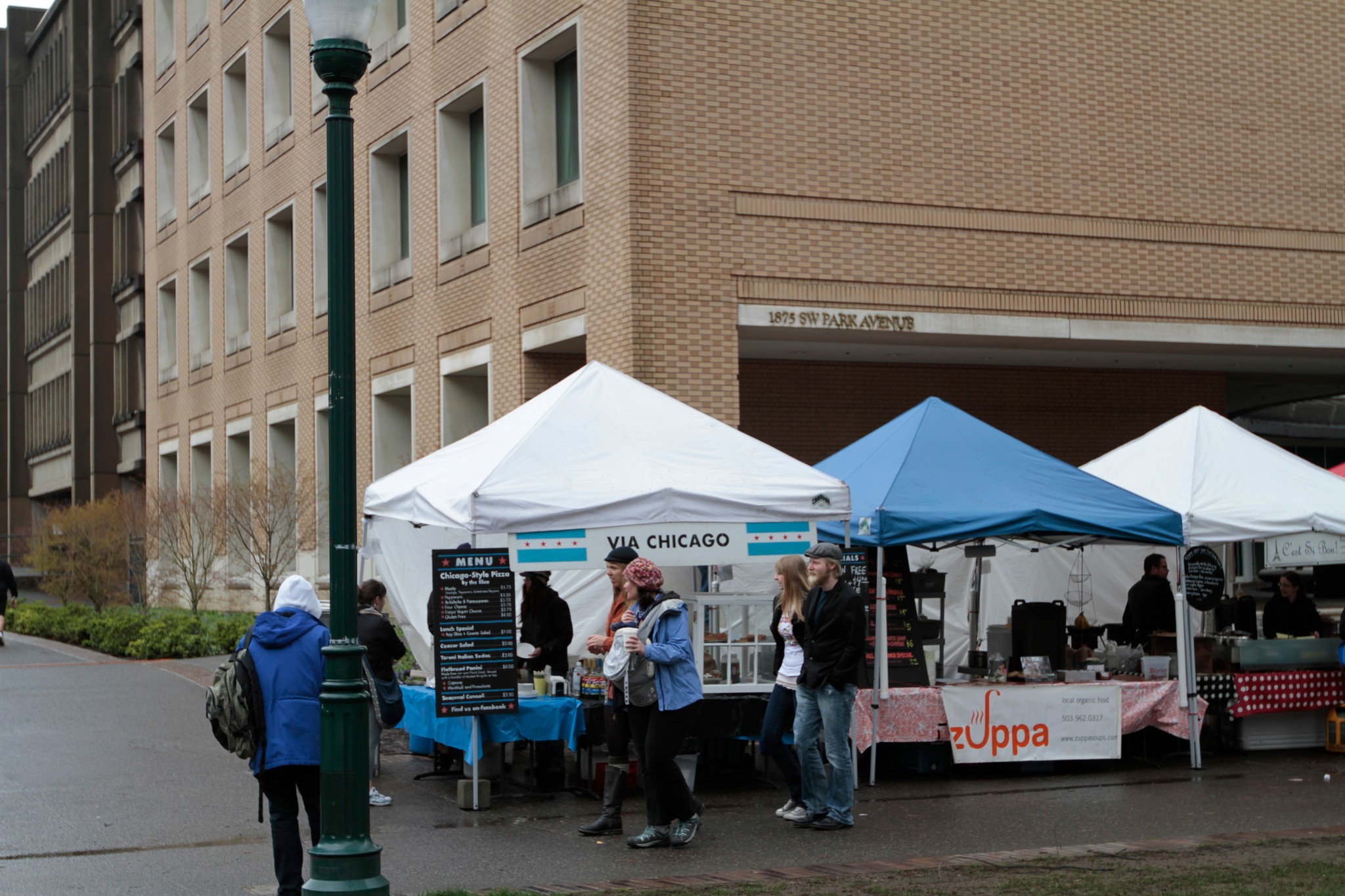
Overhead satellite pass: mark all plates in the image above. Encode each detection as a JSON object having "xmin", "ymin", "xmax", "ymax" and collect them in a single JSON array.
[{"xmin": 517, "ymin": 643, "xmax": 535, "ymax": 659}]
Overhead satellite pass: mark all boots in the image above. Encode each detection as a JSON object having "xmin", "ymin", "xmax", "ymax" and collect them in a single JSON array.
[{"xmin": 578, "ymin": 764, "xmax": 627, "ymax": 836}]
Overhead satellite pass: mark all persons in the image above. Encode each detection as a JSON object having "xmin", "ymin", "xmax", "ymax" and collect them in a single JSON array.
[
  {"xmin": 1262, "ymin": 571, "xmax": 1328, "ymax": 639},
  {"xmin": 756, "ymin": 554, "xmax": 809, "ymax": 822},
  {"xmin": 0, "ymin": 555, "xmax": 18, "ymax": 646},
  {"xmin": 608, "ymin": 557, "xmax": 705, "ymax": 849},
  {"xmin": 1122, "ymin": 553, "xmax": 1176, "ymax": 654},
  {"xmin": 518, "ymin": 571, "xmax": 573, "ymax": 790},
  {"xmin": 580, "ymin": 546, "xmax": 640, "ymax": 834},
  {"xmin": 234, "ymin": 574, "xmax": 331, "ymax": 896},
  {"xmin": 356, "ymin": 579, "xmax": 409, "ymax": 806},
  {"xmin": 794, "ymin": 542, "xmax": 866, "ymax": 830},
  {"xmin": 428, "ymin": 543, "xmax": 472, "ymax": 634}
]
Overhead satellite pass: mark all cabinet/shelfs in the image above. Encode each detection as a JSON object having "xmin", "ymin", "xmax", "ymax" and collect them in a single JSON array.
[
  {"xmin": 677, "ymin": 592, "xmax": 777, "ymax": 694},
  {"xmin": 909, "ymin": 593, "xmax": 947, "ymax": 679}
]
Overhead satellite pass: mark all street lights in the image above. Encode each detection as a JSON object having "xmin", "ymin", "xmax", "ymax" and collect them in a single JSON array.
[{"xmin": 302, "ymin": 0, "xmax": 389, "ymax": 896}]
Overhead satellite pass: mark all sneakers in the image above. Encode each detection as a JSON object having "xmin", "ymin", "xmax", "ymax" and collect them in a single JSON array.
[
  {"xmin": 368, "ymin": 786, "xmax": 392, "ymax": 807},
  {"xmin": 783, "ymin": 807, "xmax": 809, "ymax": 820},
  {"xmin": 775, "ymin": 799, "xmax": 799, "ymax": 817},
  {"xmin": 670, "ymin": 812, "xmax": 703, "ymax": 847},
  {"xmin": 628, "ymin": 824, "xmax": 670, "ymax": 847}
]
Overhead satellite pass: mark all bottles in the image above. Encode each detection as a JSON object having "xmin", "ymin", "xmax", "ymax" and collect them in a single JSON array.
[
  {"xmin": 721, "ymin": 651, "xmax": 739, "ymax": 683},
  {"xmin": 571, "ymin": 658, "xmax": 606, "ymax": 701},
  {"xmin": 1076, "ymin": 644, "xmax": 1092, "ymax": 670},
  {"xmin": 1066, "ymin": 644, "xmax": 1075, "ymax": 670}
]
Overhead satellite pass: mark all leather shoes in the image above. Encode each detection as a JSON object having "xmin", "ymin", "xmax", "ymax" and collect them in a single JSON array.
[
  {"xmin": 794, "ymin": 812, "xmax": 829, "ymax": 828},
  {"xmin": 837, "ymin": 817, "xmax": 852, "ymax": 828}
]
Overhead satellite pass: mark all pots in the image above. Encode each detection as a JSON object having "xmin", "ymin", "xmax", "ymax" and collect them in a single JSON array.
[
  {"xmin": 967, "ymin": 650, "xmax": 986, "ymax": 669},
  {"xmin": 1214, "ymin": 630, "xmax": 1249, "ymax": 665},
  {"xmin": 1067, "ymin": 624, "xmax": 1105, "ymax": 635}
]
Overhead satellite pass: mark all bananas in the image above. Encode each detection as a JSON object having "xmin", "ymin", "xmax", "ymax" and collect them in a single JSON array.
[{"xmin": 1075, "ymin": 611, "xmax": 1090, "ymax": 627}]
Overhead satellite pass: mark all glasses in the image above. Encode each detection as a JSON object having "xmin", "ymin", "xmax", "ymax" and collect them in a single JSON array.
[
  {"xmin": 1278, "ymin": 583, "xmax": 1296, "ymax": 588},
  {"xmin": 1158, "ymin": 565, "xmax": 1168, "ymax": 570}
]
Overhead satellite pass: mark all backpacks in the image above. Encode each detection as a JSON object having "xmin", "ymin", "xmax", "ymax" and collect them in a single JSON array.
[
  {"xmin": 603, "ymin": 627, "xmax": 659, "ymax": 709},
  {"xmin": 204, "ymin": 618, "xmax": 264, "ymax": 761}
]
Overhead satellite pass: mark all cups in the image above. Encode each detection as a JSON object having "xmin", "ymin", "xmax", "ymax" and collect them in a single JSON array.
[
  {"xmin": 518, "ymin": 683, "xmax": 533, "ymax": 694},
  {"xmin": 534, "ymin": 675, "xmax": 547, "ymax": 696},
  {"xmin": 623, "ymin": 626, "xmax": 639, "ymax": 651}
]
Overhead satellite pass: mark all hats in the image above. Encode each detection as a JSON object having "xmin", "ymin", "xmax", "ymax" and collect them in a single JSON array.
[
  {"xmin": 519, "ymin": 571, "xmax": 551, "ymax": 585},
  {"xmin": 804, "ymin": 542, "xmax": 842, "ymax": 559},
  {"xmin": 622, "ymin": 557, "xmax": 663, "ymax": 589},
  {"xmin": 604, "ymin": 546, "xmax": 639, "ymax": 564}
]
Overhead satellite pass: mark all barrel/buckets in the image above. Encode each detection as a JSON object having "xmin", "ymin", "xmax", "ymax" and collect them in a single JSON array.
[{"xmin": 987, "ymin": 624, "xmax": 1010, "ymax": 669}]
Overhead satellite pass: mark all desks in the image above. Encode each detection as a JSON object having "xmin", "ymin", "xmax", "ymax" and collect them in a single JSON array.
[
  {"xmin": 400, "ymin": 682, "xmax": 579, "ymax": 800},
  {"xmin": 568, "ymin": 690, "xmax": 774, "ymax": 802},
  {"xmin": 848, "ymin": 678, "xmax": 1207, "ymax": 789}
]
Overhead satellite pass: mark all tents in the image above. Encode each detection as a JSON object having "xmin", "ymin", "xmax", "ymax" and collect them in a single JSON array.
[
  {"xmin": 366, "ymin": 360, "xmax": 851, "ymax": 813},
  {"xmin": 903, "ymin": 406, "xmax": 1345, "ymax": 771},
  {"xmin": 810, "ymin": 396, "xmax": 1196, "ymax": 793}
]
